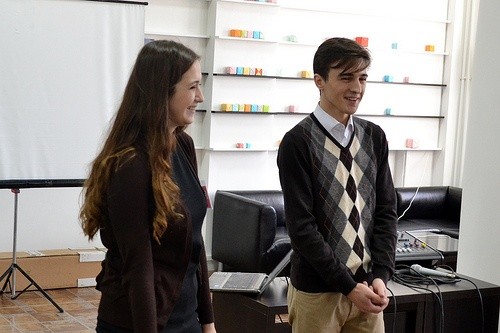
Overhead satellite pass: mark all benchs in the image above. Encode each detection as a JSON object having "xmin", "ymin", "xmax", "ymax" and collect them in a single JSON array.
[{"xmin": 212, "ymin": 186, "xmax": 463, "ymax": 273}]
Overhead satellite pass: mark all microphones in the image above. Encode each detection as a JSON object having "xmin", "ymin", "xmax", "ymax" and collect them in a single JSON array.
[{"xmin": 410, "ymin": 264, "xmax": 460, "ymax": 279}]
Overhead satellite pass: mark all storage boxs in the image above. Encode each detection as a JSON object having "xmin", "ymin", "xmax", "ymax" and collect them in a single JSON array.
[{"xmin": 0, "ymin": 249, "xmax": 108, "ymax": 292}]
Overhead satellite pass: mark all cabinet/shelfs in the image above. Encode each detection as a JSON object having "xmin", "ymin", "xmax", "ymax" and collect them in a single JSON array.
[{"xmin": 144, "ymin": 0, "xmax": 451, "ymax": 152}]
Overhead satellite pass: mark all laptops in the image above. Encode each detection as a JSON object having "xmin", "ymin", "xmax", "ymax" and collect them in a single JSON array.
[{"xmin": 209, "ymin": 248, "xmax": 294, "ymax": 295}]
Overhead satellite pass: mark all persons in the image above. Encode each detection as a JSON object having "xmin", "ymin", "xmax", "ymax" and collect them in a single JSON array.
[
  {"xmin": 277, "ymin": 37, "xmax": 399, "ymax": 333},
  {"xmin": 78, "ymin": 39, "xmax": 217, "ymax": 333}
]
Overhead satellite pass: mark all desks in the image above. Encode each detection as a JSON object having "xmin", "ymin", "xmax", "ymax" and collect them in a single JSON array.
[{"xmin": 212, "ymin": 265, "xmax": 500, "ymax": 333}]
